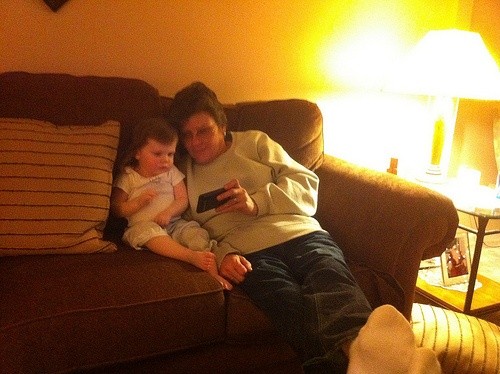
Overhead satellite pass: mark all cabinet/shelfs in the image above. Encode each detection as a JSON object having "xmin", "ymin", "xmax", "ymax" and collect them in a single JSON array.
[{"xmin": 415, "ymin": 208, "xmax": 500, "ymax": 316}]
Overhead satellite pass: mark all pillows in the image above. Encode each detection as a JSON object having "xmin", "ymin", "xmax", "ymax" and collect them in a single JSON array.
[
  {"xmin": 410, "ymin": 303, "xmax": 500, "ymax": 374},
  {"xmin": 0, "ymin": 118, "xmax": 121, "ymax": 254}
]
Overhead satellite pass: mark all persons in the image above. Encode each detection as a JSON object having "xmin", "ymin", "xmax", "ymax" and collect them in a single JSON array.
[
  {"xmin": 111, "ymin": 117, "xmax": 232, "ymax": 290},
  {"xmin": 169, "ymin": 81, "xmax": 441, "ymax": 374}
]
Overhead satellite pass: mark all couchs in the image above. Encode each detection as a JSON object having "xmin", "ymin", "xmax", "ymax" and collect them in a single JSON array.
[{"xmin": 0, "ymin": 70, "xmax": 459, "ymax": 374}]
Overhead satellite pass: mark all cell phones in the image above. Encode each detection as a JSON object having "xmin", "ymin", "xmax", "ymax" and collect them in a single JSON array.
[{"xmin": 196, "ymin": 187, "xmax": 230, "ymax": 213}]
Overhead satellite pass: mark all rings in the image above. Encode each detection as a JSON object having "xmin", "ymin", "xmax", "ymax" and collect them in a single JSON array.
[{"xmin": 233, "ymin": 198, "xmax": 239, "ymax": 202}]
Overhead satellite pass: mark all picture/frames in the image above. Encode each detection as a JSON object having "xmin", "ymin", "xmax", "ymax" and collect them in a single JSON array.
[{"xmin": 441, "ymin": 231, "xmax": 472, "ymax": 287}]
